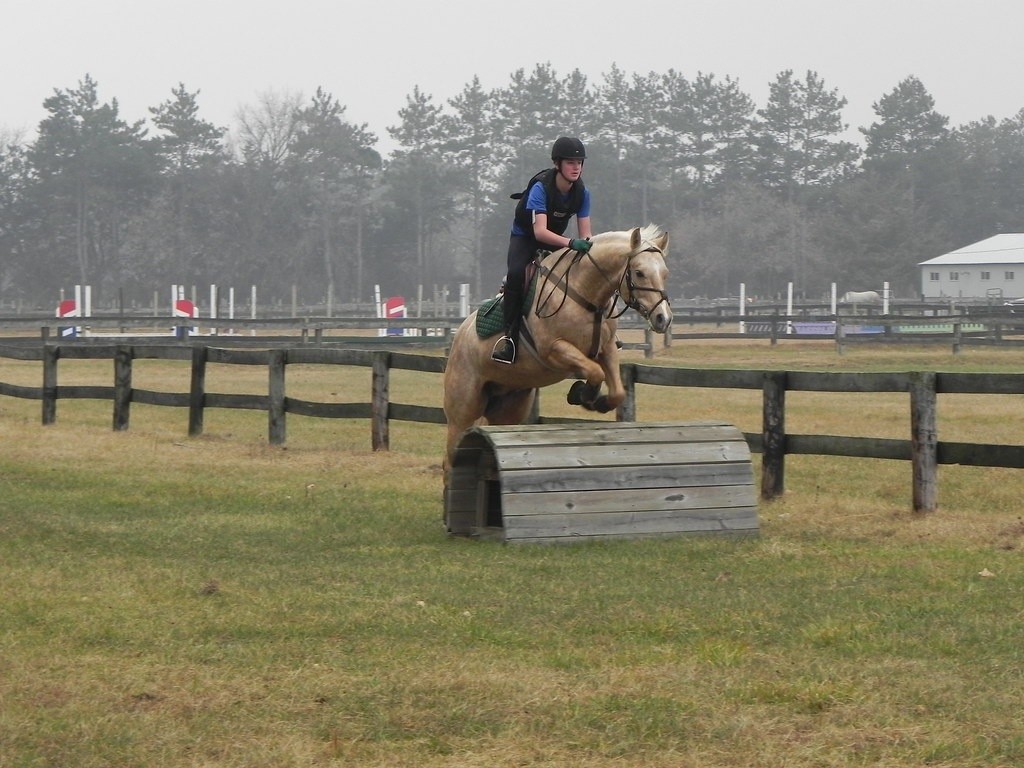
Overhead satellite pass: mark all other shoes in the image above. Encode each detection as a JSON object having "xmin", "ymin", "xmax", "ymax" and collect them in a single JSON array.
[{"xmin": 491, "ymin": 344, "xmax": 514, "ymax": 363}]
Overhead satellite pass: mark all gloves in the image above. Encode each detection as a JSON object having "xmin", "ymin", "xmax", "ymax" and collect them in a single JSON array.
[{"xmin": 569, "ymin": 239, "xmax": 593, "ymax": 252}]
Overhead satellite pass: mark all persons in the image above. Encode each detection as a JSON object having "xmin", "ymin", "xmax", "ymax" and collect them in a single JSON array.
[{"xmin": 491, "ymin": 136, "xmax": 625, "ymax": 363}]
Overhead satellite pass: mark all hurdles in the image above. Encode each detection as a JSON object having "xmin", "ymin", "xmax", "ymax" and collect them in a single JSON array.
[{"xmin": 442, "ymin": 423, "xmax": 759, "ymax": 542}]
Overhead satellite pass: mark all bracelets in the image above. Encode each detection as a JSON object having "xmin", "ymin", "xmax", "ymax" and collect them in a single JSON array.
[{"xmin": 568, "ymin": 239, "xmax": 574, "ymax": 248}]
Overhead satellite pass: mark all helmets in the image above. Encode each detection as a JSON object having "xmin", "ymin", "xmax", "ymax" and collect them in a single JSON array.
[{"xmin": 551, "ymin": 137, "xmax": 588, "ymax": 160}]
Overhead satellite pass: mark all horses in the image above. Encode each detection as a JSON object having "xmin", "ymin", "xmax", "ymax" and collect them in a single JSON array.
[
  {"xmin": 840, "ymin": 291, "xmax": 880, "ymax": 303},
  {"xmin": 442, "ymin": 222, "xmax": 673, "ymax": 528}
]
